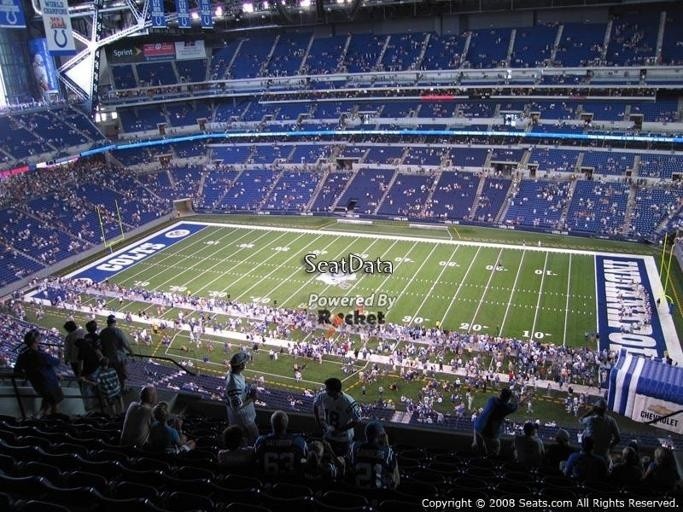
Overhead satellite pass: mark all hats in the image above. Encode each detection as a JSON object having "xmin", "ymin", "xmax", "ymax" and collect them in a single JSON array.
[
  {"xmin": 152, "ymin": 401, "xmax": 168, "ymax": 419},
  {"xmin": 594, "ymin": 399, "xmax": 606, "ymax": 409},
  {"xmin": 554, "ymin": 429, "xmax": 568, "ymax": 441},
  {"xmin": 230, "ymin": 352, "xmax": 249, "ymax": 367},
  {"xmin": 523, "ymin": 423, "xmax": 538, "ymax": 432}
]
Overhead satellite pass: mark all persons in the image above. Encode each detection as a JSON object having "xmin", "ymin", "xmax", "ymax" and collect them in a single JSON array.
[{"xmin": 0, "ymin": 13, "xmax": 683, "ymax": 512}]
